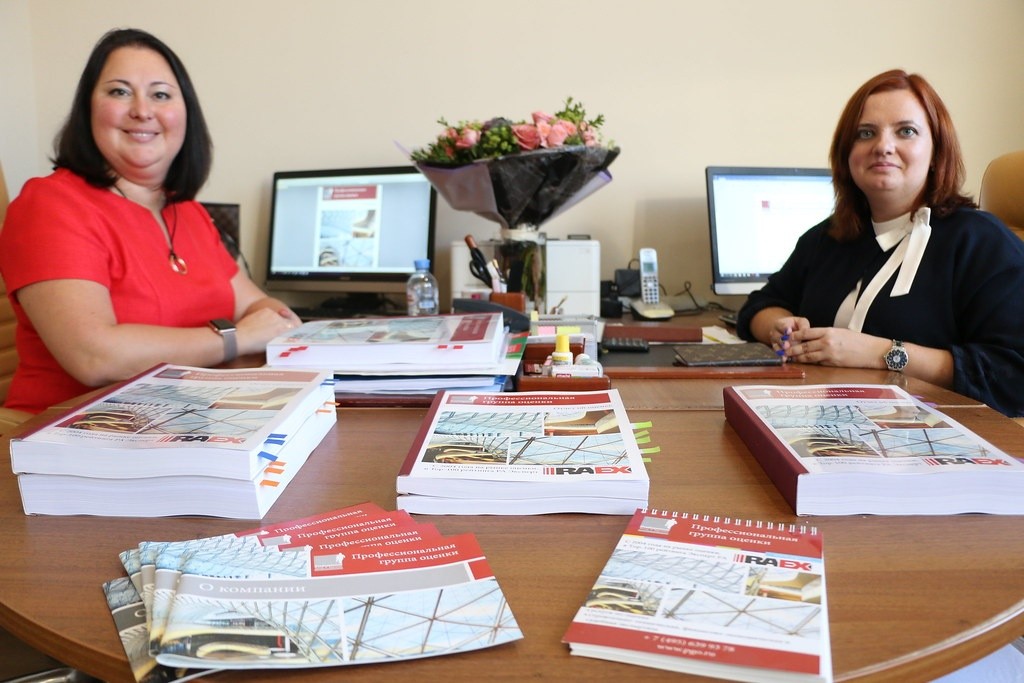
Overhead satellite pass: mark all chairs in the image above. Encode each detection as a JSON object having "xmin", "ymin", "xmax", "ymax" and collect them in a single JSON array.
[
  {"xmin": 981, "ymin": 149, "xmax": 1024, "ymax": 427},
  {"xmin": 0, "ymin": 156, "xmax": 35, "ymax": 437}
]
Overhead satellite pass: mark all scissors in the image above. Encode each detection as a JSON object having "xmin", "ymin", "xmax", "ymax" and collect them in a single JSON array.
[{"xmin": 469, "ymin": 248, "xmax": 491, "ymax": 288}]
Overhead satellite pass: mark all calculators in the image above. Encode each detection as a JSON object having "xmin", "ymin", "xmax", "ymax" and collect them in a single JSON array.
[{"xmin": 602, "ymin": 338, "xmax": 649, "ymax": 351}]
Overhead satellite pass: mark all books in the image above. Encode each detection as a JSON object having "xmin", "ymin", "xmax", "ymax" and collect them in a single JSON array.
[
  {"xmin": 560, "ymin": 508, "xmax": 834, "ymax": 683},
  {"xmin": 99, "ymin": 502, "xmax": 524, "ymax": 682},
  {"xmin": 267, "ymin": 311, "xmax": 507, "ymax": 395},
  {"xmin": 723, "ymin": 383, "xmax": 1024, "ymax": 517},
  {"xmin": 396, "ymin": 389, "xmax": 650, "ymax": 515},
  {"xmin": 672, "ymin": 343, "xmax": 782, "ymax": 368},
  {"xmin": 9, "ymin": 363, "xmax": 337, "ymax": 519}
]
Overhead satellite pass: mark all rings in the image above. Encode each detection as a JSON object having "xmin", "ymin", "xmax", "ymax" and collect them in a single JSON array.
[{"xmin": 772, "ymin": 343, "xmax": 777, "ymax": 348}]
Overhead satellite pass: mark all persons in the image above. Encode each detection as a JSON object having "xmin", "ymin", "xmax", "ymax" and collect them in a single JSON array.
[
  {"xmin": 0, "ymin": 29, "xmax": 302, "ymax": 416},
  {"xmin": 736, "ymin": 69, "xmax": 1024, "ymax": 418}
]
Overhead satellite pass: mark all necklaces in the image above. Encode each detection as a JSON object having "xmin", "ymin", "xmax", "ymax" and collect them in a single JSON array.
[{"xmin": 114, "ymin": 187, "xmax": 188, "ymax": 276}]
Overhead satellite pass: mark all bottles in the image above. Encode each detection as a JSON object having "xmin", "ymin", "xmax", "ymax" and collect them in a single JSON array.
[
  {"xmin": 405, "ymin": 258, "xmax": 440, "ymax": 316},
  {"xmin": 551, "ymin": 335, "xmax": 574, "ymax": 365}
]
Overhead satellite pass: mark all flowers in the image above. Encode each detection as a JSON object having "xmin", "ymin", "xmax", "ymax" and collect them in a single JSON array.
[{"xmin": 402, "ymin": 96, "xmax": 620, "ymax": 296}]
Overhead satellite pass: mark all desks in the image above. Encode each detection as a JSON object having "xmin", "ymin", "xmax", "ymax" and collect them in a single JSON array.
[{"xmin": 0, "ymin": 304, "xmax": 1024, "ymax": 683}]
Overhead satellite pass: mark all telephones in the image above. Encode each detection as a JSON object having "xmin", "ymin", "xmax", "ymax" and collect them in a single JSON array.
[{"xmin": 632, "ymin": 248, "xmax": 675, "ymax": 320}]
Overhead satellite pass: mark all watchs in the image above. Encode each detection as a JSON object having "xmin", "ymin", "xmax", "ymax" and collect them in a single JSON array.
[
  {"xmin": 208, "ymin": 318, "xmax": 238, "ymax": 362},
  {"xmin": 879, "ymin": 371, "xmax": 907, "ymax": 388},
  {"xmin": 884, "ymin": 339, "xmax": 908, "ymax": 371}
]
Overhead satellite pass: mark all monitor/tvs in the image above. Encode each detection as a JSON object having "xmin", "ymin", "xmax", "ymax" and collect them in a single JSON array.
[
  {"xmin": 705, "ymin": 166, "xmax": 839, "ymax": 294},
  {"xmin": 266, "ymin": 165, "xmax": 438, "ymax": 318}
]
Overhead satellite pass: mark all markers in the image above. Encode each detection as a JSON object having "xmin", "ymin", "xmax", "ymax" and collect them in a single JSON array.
[{"xmin": 464, "ymin": 235, "xmax": 483, "ymax": 263}]
